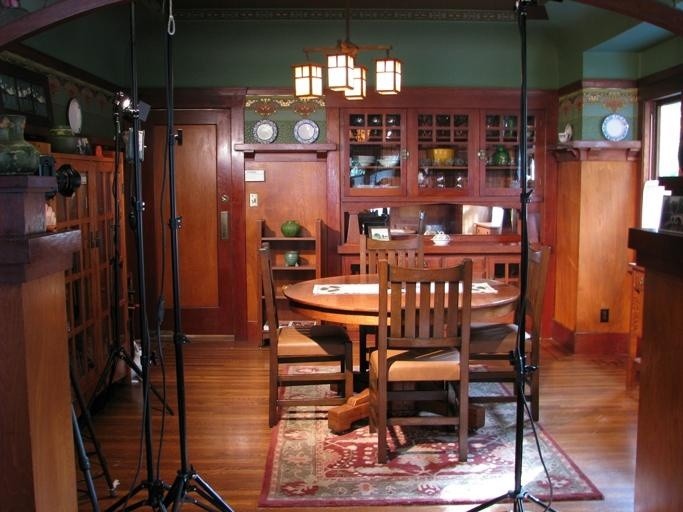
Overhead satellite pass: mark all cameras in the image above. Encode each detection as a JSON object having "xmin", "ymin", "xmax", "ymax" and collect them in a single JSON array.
[{"xmin": 35, "ymin": 151, "xmax": 81, "ymax": 200}]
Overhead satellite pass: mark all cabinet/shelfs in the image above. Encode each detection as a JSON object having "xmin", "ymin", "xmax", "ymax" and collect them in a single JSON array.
[
  {"xmin": 324, "ymin": 88, "xmax": 559, "ymax": 339},
  {"xmin": 256, "ymin": 219, "xmax": 322, "ymax": 348},
  {"xmin": 40, "ymin": 151, "xmax": 131, "ymax": 420}
]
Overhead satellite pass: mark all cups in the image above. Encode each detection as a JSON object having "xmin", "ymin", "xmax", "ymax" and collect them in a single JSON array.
[
  {"xmin": 558, "ymin": 133, "xmax": 567, "ymax": 143},
  {"xmin": 435, "ymin": 170, "xmax": 465, "ymax": 190},
  {"xmin": 430, "ymin": 231, "xmax": 453, "ymax": 245},
  {"xmin": 353, "ymin": 115, "xmax": 396, "ymax": 140}
]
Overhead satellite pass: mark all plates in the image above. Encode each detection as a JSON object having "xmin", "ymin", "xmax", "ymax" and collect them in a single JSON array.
[
  {"xmin": 567, "ymin": 123, "xmax": 572, "ymax": 139},
  {"xmin": 389, "ymin": 229, "xmax": 415, "ymax": 239},
  {"xmin": 66, "ymin": 96, "xmax": 83, "ymax": 135},
  {"xmin": 601, "ymin": 114, "xmax": 630, "ymax": 141},
  {"xmin": 293, "ymin": 119, "xmax": 318, "ymax": 144},
  {"xmin": 251, "ymin": 119, "xmax": 279, "ymax": 144}
]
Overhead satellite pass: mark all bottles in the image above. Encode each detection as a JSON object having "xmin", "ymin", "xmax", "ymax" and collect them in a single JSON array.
[{"xmin": 494, "ymin": 145, "xmax": 509, "ymax": 165}]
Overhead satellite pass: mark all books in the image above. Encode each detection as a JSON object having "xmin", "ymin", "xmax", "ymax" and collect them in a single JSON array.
[{"xmin": 263, "ymin": 318, "xmax": 316, "ymax": 331}]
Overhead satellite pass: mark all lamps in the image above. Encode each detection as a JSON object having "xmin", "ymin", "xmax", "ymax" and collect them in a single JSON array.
[{"xmin": 290, "ymin": 1, "xmax": 403, "ymax": 101}]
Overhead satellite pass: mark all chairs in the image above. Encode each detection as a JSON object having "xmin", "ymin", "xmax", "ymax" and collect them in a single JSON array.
[
  {"xmin": 359, "ymin": 233, "xmax": 425, "ymax": 391},
  {"xmin": 451, "ymin": 245, "xmax": 551, "ymax": 421},
  {"xmin": 259, "ymin": 242, "xmax": 353, "ymax": 428},
  {"xmin": 368, "ymin": 259, "xmax": 473, "ymax": 464}
]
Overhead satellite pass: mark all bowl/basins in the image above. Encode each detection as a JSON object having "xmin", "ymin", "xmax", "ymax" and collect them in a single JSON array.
[
  {"xmin": 351, "ymin": 154, "xmax": 374, "ymax": 168},
  {"xmin": 377, "ymin": 156, "xmax": 400, "ymax": 168}
]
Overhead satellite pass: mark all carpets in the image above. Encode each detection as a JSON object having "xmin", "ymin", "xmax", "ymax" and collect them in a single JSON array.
[{"xmin": 258, "ymin": 365, "xmax": 605, "ymax": 502}]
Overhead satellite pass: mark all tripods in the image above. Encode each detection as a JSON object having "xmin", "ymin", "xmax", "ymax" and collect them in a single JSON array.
[
  {"xmin": 156, "ymin": 0, "xmax": 236, "ymax": 512},
  {"xmin": 78, "ymin": 108, "xmax": 174, "ymax": 432},
  {"xmin": 103, "ymin": 0, "xmax": 218, "ymax": 512},
  {"xmin": 465, "ymin": 0, "xmax": 558, "ymax": 512}
]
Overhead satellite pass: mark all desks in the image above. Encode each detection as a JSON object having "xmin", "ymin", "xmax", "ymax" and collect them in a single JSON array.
[
  {"xmin": 283, "ymin": 274, "xmax": 521, "ymax": 327},
  {"xmin": 625, "ymin": 260, "xmax": 646, "ymax": 389}
]
